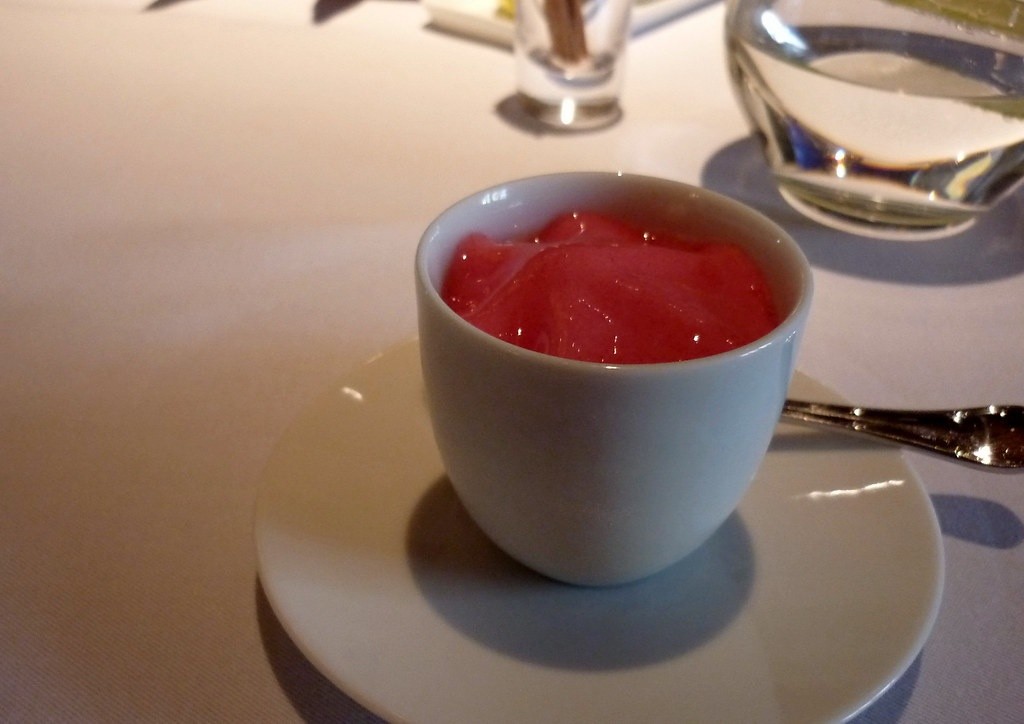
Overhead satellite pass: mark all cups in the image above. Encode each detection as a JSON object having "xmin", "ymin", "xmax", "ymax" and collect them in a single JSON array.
[
  {"xmin": 725, "ymin": 1, "xmax": 1024, "ymax": 241},
  {"xmin": 415, "ymin": 170, "xmax": 815, "ymax": 592},
  {"xmin": 512, "ymin": 0, "xmax": 635, "ymax": 130}
]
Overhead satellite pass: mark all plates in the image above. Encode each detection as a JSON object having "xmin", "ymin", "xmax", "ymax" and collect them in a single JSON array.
[
  {"xmin": 251, "ymin": 338, "xmax": 945, "ymax": 724},
  {"xmin": 421, "ymin": 0, "xmax": 720, "ymax": 51}
]
